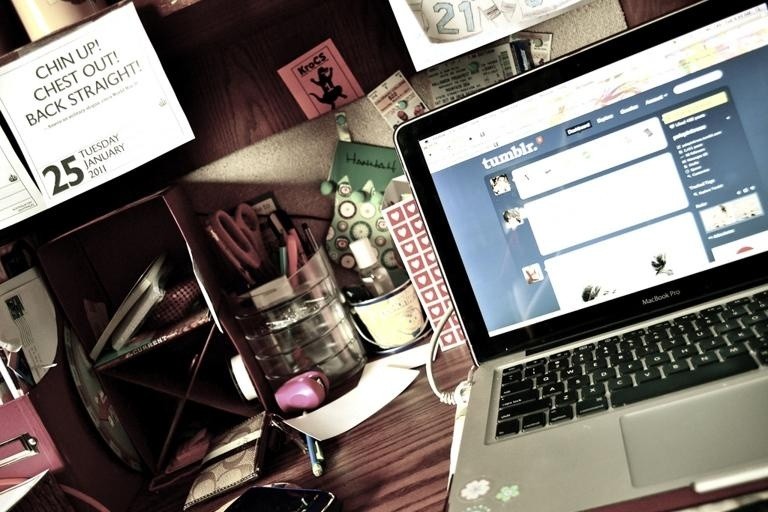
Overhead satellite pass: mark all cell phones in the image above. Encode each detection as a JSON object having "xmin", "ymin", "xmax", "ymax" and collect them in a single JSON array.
[{"xmin": 224, "ymin": 485, "xmax": 335, "ymax": 512}]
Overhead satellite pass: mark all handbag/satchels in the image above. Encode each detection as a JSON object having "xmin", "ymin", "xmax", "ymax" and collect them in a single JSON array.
[{"xmin": 184, "ymin": 411, "xmax": 308, "ymax": 508}]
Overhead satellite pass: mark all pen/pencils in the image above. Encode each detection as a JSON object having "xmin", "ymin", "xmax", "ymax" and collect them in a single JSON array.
[
  {"xmin": 203, "ymin": 223, "xmax": 255, "ymax": 296},
  {"xmin": 266, "ymin": 210, "xmax": 359, "ymax": 370},
  {"xmin": 300, "ymin": 411, "xmax": 322, "ymax": 477}
]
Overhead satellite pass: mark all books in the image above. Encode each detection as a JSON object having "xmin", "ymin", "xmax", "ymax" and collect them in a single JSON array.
[{"xmin": 88, "ymin": 249, "xmax": 172, "ymax": 362}]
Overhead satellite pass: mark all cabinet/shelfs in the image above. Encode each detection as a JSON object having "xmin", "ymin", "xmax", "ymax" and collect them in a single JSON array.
[
  {"xmin": 36, "ymin": 181, "xmax": 274, "ymax": 476},
  {"xmin": 0, "ymin": 235, "xmax": 148, "ymax": 512}
]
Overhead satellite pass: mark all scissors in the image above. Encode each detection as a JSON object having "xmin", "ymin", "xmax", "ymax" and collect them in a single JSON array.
[{"xmin": 214, "ymin": 204, "xmax": 279, "ymax": 286}]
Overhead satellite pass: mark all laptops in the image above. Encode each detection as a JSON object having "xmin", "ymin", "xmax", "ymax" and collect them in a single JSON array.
[{"xmin": 392, "ymin": 0, "xmax": 768, "ymax": 512}]
